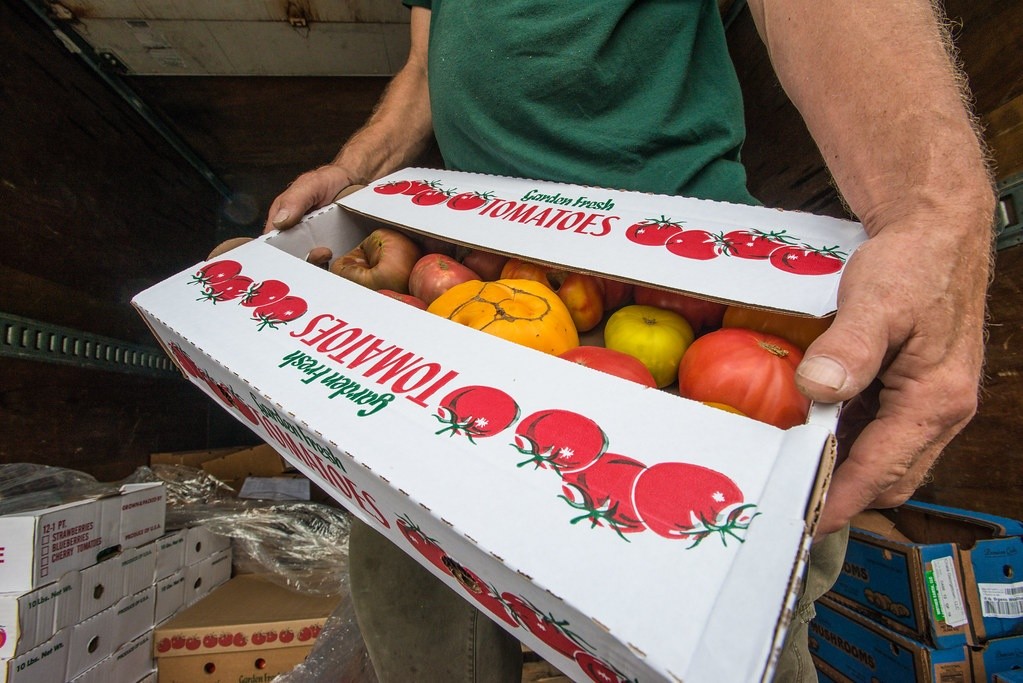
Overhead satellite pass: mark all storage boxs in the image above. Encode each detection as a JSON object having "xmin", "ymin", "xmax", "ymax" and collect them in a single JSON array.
[
  {"xmin": 808, "ymin": 500, "xmax": 1023, "ymax": 683},
  {"xmin": 0, "ymin": 443, "xmax": 353, "ymax": 683},
  {"xmin": 132, "ymin": 168, "xmax": 869, "ymax": 683}
]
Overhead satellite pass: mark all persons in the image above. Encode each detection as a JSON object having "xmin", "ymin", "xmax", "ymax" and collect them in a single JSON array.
[{"xmin": 264, "ymin": 0, "xmax": 998, "ymax": 683}]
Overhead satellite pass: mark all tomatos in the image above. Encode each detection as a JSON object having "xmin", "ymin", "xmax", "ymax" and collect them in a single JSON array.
[{"xmin": 306, "ymin": 230, "xmax": 836, "ymax": 430}]
